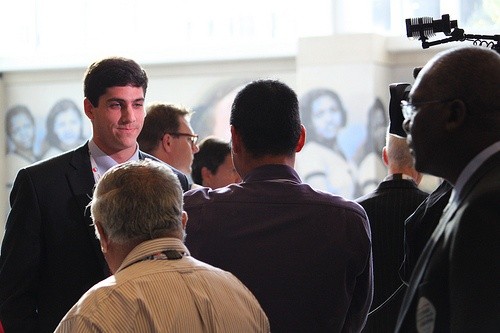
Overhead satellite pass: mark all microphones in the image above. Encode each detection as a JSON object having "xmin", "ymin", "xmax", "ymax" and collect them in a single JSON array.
[{"xmin": 406, "ymin": 15, "xmax": 457, "ymax": 38}]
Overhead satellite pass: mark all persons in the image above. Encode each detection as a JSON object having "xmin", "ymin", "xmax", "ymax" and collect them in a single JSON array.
[
  {"xmin": 182, "ymin": 80, "xmax": 374, "ymax": 333},
  {"xmin": 41, "ymin": 99, "xmax": 86, "ymax": 160},
  {"xmin": 5, "ymin": 105, "xmax": 41, "ymax": 185},
  {"xmin": 136, "ymin": 105, "xmax": 199, "ymax": 173},
  {"xmin": 192, "ymin": 138, "xmax": 242, "ymax": 189},
  {"xmin": 360, "ymin": 47, "xmax": 500, "ymax": 333},
  {"xmin": 0, "ymin": 57, "xmax": 188, "ymax": 333},
  {"xmin": 357, "ymin": 97, "xmax": 389, "ymax": 195},
  {"xmin": 54, "ymin": 158, "xmax": 270, "ymax": 333},
  {"xmin": 354, "ymin": 123, "xmax": 433, "ymax": 314},
  {"xmin": 295, "ymin": 88, "xmax": 358, "ymax": 200}
]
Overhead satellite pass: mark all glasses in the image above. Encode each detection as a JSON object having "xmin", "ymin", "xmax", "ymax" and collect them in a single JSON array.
[
  {"xmin": 400, "ymin": 99, "xmax": 457, "ymax": 117},
  {"xmin": 170, "ymin": 132, "xmax": 198, "ymax": 143}
]
